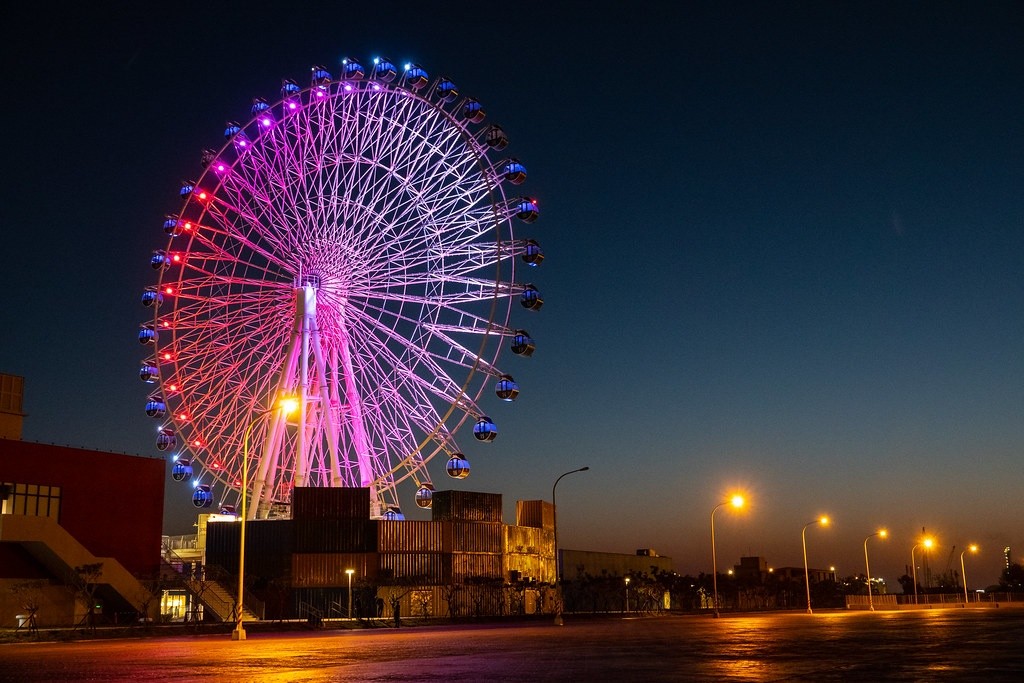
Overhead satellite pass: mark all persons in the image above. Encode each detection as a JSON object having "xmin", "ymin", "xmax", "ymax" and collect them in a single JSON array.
[
  {"xmin": 355, "ymin": 596, "xmax": 362, "ymax": 619},
  {"xmin": 393, "ymin": 601, "xmax": 400, "ymax": 628},
  {"xmin": 376, "ymin": 598, "xmax": 384, "ymax": 618}
]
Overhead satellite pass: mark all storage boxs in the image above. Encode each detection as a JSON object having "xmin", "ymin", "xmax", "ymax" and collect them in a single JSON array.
[{"xmin": 203, "ymin": 484, "xmax": 556, "ymax": 623}]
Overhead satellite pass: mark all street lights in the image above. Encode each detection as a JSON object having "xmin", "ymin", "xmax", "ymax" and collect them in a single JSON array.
[
  {"xmin": 912, "ymin": 541, "xmax": 932, "ymax": 604},
  {"xmin": 961, "ymin": 547, "xmax": 976, "ymax": 603},
  {"xmin": 232, "ymin": 402, "xmax": 298, "ymax": 642},
  {"xmin": 346, "ymin": 570, "xmax": 354, "ymax": 618},
  {"xmin": 712, "ymin": 498, "xmax": 742, "ymax": 618},
  {"xmin": 553, "ymin": 466, "xmax": 590, "ymax": 626},
  {"xmin": 626, "ymin": 579, "xmax": 630, "ymax": 611},
  {"xmin": 802, "ymin": 519, "xmax": 827, "ymax": 614},
  {"xmin": 864, "ymin": 531, "xmax": 886, "ymax": 611}
]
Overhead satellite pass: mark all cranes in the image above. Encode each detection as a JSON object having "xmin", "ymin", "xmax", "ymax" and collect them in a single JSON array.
[{"xmin": 922, "ymin": 527, "xmax": 955, "ymax": 588}]
